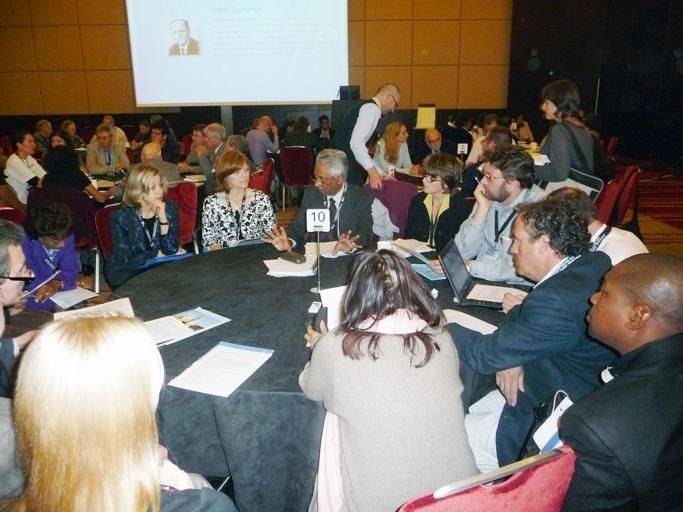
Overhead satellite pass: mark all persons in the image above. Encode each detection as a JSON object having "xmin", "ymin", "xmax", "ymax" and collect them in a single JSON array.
[
  {"xmin": 1, "ymin": 110, "xmax": 683, "ymax": 512},
  {"xmin": 327, "ymin": 82, "xmax": 400, "ymax": 189},
  {"xmin": 533, "ymin": 79, "xmax": 603, "ymax": 192},
  {"xmin": 167, "ymin": 18, "xmax": 198, "ymax": 55}
]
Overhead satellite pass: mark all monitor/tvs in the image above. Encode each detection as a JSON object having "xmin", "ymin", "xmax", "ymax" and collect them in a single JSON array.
[{"xmin": 340, "ymin": 85, "xmax": 360, "ymax": 100}]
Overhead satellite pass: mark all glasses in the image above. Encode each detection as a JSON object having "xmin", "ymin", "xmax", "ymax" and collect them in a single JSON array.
[
  {"xmin": 0, "ymin": 269, "xmax": 37, "ymax": 287},
  {"xmin": 310, "ymin": 175, "xmax": 335, "ymax": 185},
  {"xmin": 422, "ymin": 175, "xmax": 441, "ymax": 183},
  {"xmin": 484, "ymin": 174, "xmax": 504, "ymax": 183}
]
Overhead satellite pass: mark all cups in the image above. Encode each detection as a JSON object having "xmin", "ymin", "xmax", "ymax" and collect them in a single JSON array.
[
  {"xmin": 531, "ymin": 142, "xmax": 537, "ymax": 153},
  {"xmin": 387, "ymin": 168, "xmax": 396, "ymax": 176}
]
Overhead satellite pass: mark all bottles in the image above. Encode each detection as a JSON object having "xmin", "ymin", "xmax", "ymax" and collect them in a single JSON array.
[{"xmin": 472, "ymin": 128, "xmax": 487, "ymax": 139}]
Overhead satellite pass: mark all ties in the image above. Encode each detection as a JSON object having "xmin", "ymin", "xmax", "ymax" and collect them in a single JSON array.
[{"xmin": 328, "ymin": 197, "xmax": 338, "ymax": 224}]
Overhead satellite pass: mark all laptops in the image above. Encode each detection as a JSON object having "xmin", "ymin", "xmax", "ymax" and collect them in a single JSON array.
[{"xmin": 437, "ymin": 239, "xmax": 533, "ymax": 309}]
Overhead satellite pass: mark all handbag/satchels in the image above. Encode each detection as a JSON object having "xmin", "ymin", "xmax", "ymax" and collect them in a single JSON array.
[
  {"xmin": 536, "ymin": 160, "xmax": 605, "ymax": 205},
  {"xmin": 531, "ymin": 394, "xmax": 575, "ymax": 455},
  {"xmin": 525, "ymin": 387, "xmax": 564, "ymax": 459},
  {"xmin": 606, "ymin": 218, "xmax": 644, "ymax": 243}
]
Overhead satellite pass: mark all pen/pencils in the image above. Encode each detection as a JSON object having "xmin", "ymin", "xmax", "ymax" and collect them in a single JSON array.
[
  {"xmin": 475, "ymin": 176, "xmax": 480, "ymax": 183},
  {"xmin": 119, "ymin": 168, "xmax": 129, "ymax": 178}
]
